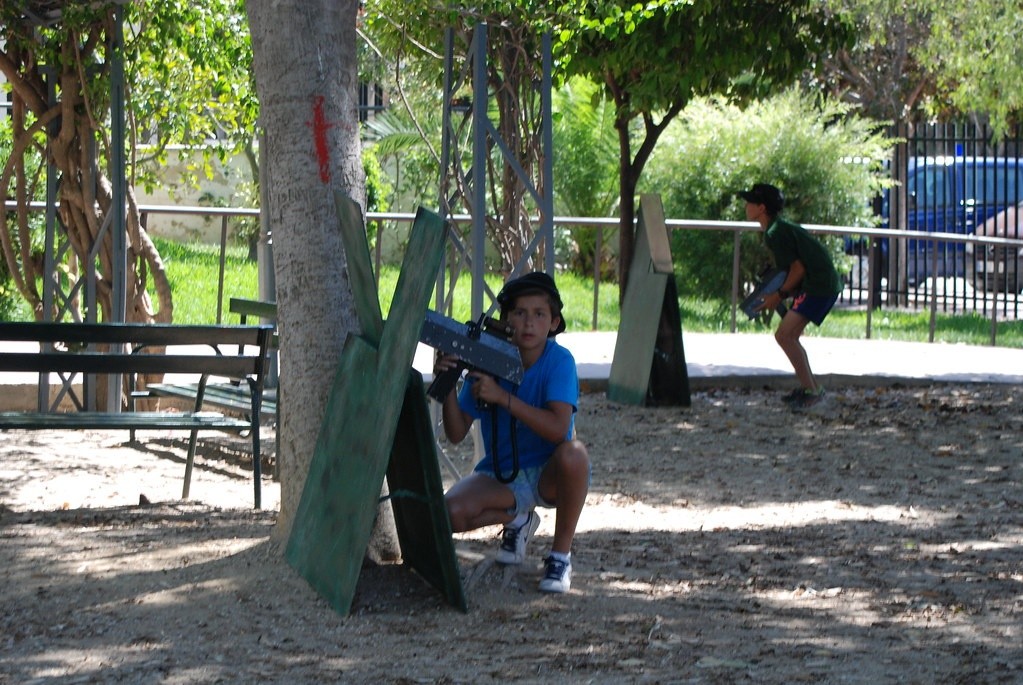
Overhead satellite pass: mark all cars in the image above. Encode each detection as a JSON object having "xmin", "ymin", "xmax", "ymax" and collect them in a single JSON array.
[{"xmin": 967, "ymin": 202, "xmax": 1023, "ymax": 298}]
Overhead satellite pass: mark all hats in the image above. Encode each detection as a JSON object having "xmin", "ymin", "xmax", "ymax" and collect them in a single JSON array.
[
  {"xmin": 496, "ymin": 271, "xmax": 566, "ymax": 337},
  {"xmin": 739, "ymin": 183, "xmax": 785, "ymax": 214}
]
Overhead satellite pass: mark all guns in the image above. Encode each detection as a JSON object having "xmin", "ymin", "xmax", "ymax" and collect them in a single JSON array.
[
  {"xmin": 417, "ymin": 303, "xmax": 526, "ymax": 412},
  {"xmin": 737, "ymin": 268, "xmax": 789, "ymax": 322}
]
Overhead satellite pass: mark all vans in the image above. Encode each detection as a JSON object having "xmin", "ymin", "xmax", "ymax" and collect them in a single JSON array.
[{"xmin": 842, "ymin": 155, "xmax": 1023, "ymax": 295}]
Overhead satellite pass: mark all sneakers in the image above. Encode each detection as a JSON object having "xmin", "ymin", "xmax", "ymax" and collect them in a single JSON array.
[
  {"xmin": 537, "ymin": 552, "xmax": 573, "ymax": 593},
  {"xmin": 781, "ymin": 384, "xmax": 826, "ymax": 404},
  {"xmin": 791, "ymin": 387, "xmax": 819, "ymax": 413},
  {"xmin": 494, "ymin": 510, "xmax": 540, "ymax": 564}
]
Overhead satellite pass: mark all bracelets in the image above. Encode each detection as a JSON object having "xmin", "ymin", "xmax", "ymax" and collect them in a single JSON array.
[{"xmin": 508, "ymin": 392, "xmax": 510, "ymax": 409}]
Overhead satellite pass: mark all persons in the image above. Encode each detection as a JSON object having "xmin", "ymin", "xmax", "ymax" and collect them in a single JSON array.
[
  {"xmin": 434, "ymin": 272, "xmax": 592, "ymax": 592},
  {"xmin": 739, "ymin": 183, "xmax": 844, "ymax": 411}
]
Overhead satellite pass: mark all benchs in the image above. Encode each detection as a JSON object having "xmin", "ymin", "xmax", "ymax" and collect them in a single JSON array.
[
  {"xmin": 0, "ymin": 322, "xmax": 274, "ymax": 509},
  {"xmin": 129, "ymin": 298, "xmax": 282, "ymax": 484}
]
